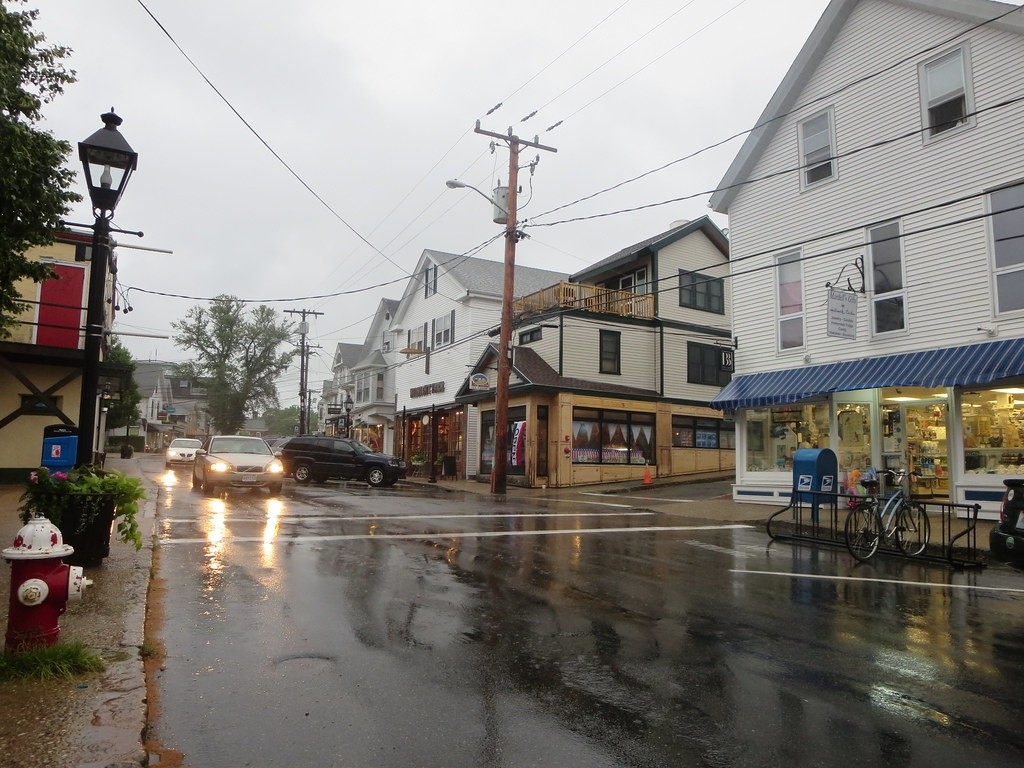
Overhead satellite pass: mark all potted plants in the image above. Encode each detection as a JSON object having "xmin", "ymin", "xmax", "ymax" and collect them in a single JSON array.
[
  {"xmin": 119, "ymin": 444, "xmax": 134, "ymax": 460},
  {"xmin": 434, "ymin": 455, "xmax": 447, "ymax": 475}
]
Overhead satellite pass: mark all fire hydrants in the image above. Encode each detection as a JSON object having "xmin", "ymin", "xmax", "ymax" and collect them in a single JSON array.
[{"xmin": 0, "ymin": 512, "xmax": 96, "ymax": 652}]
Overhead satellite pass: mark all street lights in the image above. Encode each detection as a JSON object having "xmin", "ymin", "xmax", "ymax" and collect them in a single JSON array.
[
  {"xmin": 55, "ymin": 107, "xmax": 145, "ymax": 473},
  {"xmin": 445, "ymin": 177, "xmax": 520, "ymax": 495},
  {"xmin": 282, "ymin": 364, "xmax": 309, "ymax": 436},
  {"xmin": 269, "ymin": 338, "xmax": 306, "ymax": 437},
  {"xmin": 343, "ymin": 393, "xmax": 354, "ymax": 438}
]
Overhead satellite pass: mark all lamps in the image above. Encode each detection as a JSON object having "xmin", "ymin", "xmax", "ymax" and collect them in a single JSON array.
[
  {"xmin": 110, "ymin": 400, "xmax": 114, "ymax": 408},
  {"xmin": 400, "ymin": 348, "xmax": 428, "ymax": 355},
  {"xmin": 104, "ymin": 378, "xmax": 111, "ymax": 391}
]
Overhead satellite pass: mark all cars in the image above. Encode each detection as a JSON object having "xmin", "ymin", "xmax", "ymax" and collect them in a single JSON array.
[
  {"xmin": 263, "ymin": 433, "xmax": 297, "ymax": 453},
  {"xmin": 191, "ymin": 435, "xmax": 285, "ymax": 496},
  {"xmin": 164, "ymin": 437, "xmax": 203, "ymax": 468},
  {"xmin": 988, "ymin": 477, "xmax": 1024, "ymax": 568}
]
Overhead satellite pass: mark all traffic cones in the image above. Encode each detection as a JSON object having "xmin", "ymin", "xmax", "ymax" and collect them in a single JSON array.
[{"xmin": 641, "ymin": 462, "xmax": 654, "ymax": 485}]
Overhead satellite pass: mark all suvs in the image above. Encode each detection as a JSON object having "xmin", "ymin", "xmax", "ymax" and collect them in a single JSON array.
[{"xmin": 270, "ymin": 434, "xmax": 408, "ymax": 487}]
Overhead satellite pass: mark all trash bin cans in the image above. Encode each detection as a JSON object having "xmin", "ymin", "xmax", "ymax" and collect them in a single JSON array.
[
  {"xmin": 121, "ymin": 445, "xmax": 133, "ymax": 459},
  {"xmin": 40, "ymin": 423, "xmax": 79, "ymax": 475}
]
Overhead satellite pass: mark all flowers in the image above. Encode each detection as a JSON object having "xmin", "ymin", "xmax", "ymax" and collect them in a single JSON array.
[
  {"xmin": 411, "ymin": 455, "xmax": 425, "ymax": 463},
  {"xmin": 18, "ymin": 464, "xmax": 148, "ymax": 550}
]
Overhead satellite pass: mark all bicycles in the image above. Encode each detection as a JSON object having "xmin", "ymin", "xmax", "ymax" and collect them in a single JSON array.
[{"xmin": 843, "ymin": 469, "xmax": 932, "ymax": 563}]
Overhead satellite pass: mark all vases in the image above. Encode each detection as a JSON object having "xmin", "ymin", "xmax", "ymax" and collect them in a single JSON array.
[
  {"xmin": 412, "ymin": 461, "xmax": 426, "ymax": 465},
  {"xmin": 39, "ymin": 490, "xmax": 124, "ymax": 570}
]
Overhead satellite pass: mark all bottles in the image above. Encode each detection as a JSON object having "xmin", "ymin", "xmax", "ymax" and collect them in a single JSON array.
[
  {"xmin": 920, "ymin": 458, "xmax": 935, "ymax": 475},
  {"xmin": 923, "ymin": 443, "xmax": 938, "ymax": 454}
]
